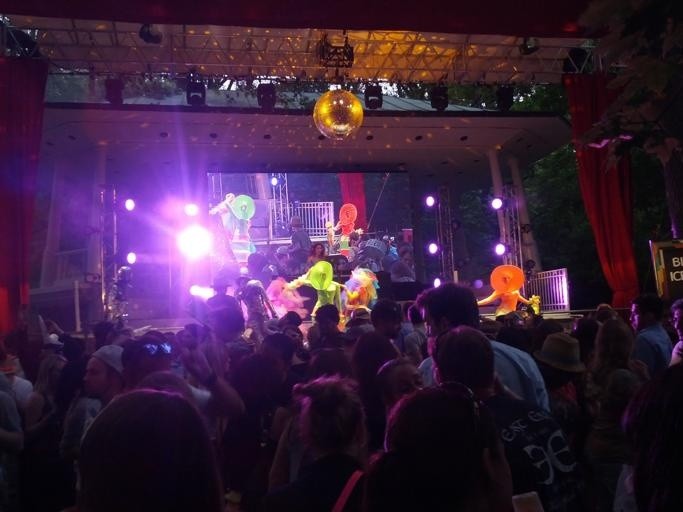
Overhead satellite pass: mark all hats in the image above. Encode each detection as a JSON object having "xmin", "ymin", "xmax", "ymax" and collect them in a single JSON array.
[
  {"xmin": 91, "ymin": 345, "xmax": 126, "ymax": 373},
  {"xmin": 534, "ymin": 331, "xmax": 589, "ymax": 376},
  {"xmin": 208, "ymin": 276, "xmax": 234, "ymax": 288},
  {"xmin": 287, "ymin": 215, "xmax": 306, "ymax": 227}
]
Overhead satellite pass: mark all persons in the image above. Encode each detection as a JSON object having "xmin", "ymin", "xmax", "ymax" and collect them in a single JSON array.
[{"xmin": 1, "ymin": 217, "xmax": 683, "ymax": 512}]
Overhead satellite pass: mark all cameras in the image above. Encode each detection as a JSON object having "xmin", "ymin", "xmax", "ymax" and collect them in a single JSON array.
[{"xmin": 141, "ymin": 341, "xmax": 175, "ymax": 357}]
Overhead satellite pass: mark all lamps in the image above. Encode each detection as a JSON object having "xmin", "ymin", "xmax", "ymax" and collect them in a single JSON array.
[
  {"xmin": 421, "ymin": 189, "xmax": 466, "ymax": 290},
  {"xmin": 132, "ymin": 20, "xmax": 168, "ymax": 46},
  {"xmin": 515, "ymin": 33, "xmax": 543, "ymax": 57},
  {"xmin": 92, "ymin": 67, "xmax": 523, "ymax": 116},
  {"xmin": 490, "ymin": 194, "xmax": 537, "ymax": 276}
]
